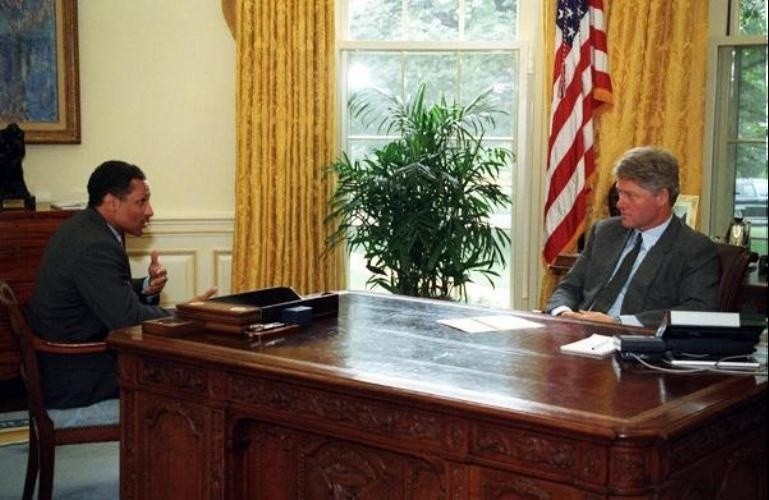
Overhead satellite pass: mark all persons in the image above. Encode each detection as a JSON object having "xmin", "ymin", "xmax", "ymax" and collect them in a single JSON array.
[
  {"xmin": 24, "ymin": 160, "xmax": 219, "ymax": 411},
  {"xmin": 545, "ymin": 144, "xmax": 725, "ymax": 330}
]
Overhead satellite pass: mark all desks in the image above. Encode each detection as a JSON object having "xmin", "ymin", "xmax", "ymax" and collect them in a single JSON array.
[{"xmin": 104, "ymin": 291, "xmax": 769, "ymax": 500}]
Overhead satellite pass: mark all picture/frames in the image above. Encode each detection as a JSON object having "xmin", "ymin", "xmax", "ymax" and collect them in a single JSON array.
[{"xmin": 0, "ymin": 0, "xmax": 82, "ymax": 145}]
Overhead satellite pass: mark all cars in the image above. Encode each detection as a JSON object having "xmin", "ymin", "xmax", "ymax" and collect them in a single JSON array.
[{"xmin": 734, "ymin": 177, "xmax": 768, "ymax": 222}]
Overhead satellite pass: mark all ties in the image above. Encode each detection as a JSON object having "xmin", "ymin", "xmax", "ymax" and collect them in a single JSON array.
[{"xmin": 591, "ymin": 234, "xmax": 643, "ymax": 313}]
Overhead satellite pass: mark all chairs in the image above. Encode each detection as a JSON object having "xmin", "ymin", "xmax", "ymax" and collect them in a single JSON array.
[
  {"xmin": 713, "ymin": 246, "xmax": 752, "ymax": 313},
  {"xmin": 0, "ymin": 281, "xmax": 121, "ymax": 500}
]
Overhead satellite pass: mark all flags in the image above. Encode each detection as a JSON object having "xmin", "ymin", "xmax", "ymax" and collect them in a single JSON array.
[{"xmin": 539, "ymin": 1, "xmax": 613, "ymax": 279}]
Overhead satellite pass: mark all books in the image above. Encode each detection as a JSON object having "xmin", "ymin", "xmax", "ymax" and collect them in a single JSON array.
[{"xmin": 557, "ymin": 332, "xmax": 621, "ymax": 359}]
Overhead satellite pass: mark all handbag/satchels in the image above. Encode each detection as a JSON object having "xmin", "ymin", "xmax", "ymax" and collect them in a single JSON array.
[{"xmin": 0, "ymin": 122, "xmax": 30, "ymax": 200}]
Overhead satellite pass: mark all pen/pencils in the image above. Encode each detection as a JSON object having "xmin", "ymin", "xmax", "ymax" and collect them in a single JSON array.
[
  {"xmin": 592, "ymin": 340, "xmax": 608, "ymax": 351},
  {"xmin": 672, "ymin": 360, "xmax": 758, "ymax": 368}
]
